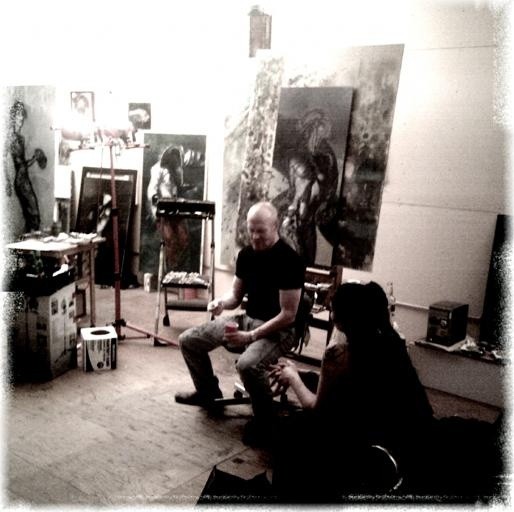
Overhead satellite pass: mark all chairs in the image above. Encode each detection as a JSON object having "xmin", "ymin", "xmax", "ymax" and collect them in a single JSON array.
[
  {"xmin": 209, "ymin": 289, "xmax": 314, "ymax": 429},
  {"xmin": 152, "ymin": 198, "xmax": 215, "ymax": 346}
]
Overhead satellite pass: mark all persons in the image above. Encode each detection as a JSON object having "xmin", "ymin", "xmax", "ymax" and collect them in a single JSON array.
[
  {"xmin": 244, "ymin": 282, "xmax": 437, "ymax": 451},
  {"xmin": 175, "ymin": 201, "xmax": 308, "ymax": 415},
  {"xmin": 146, "ymin": 144, "xmax": 193, "ymax": 273},
  {"xmin": 3, "ymin": 101, "xmax": 43, "ymax": 234}
]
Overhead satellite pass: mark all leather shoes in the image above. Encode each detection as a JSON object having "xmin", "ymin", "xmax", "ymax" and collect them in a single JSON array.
[{"xmin": 174, "ymin": 388, "xmax": 224, "ymax": 406}]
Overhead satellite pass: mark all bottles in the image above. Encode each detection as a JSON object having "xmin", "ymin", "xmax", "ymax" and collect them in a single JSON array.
[{"xmin": 386, "ymin": 281, "xmax": 396, "ymax": 324}]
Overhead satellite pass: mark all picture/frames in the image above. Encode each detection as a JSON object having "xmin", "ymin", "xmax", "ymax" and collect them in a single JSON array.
[{"xmin": 71, "ymin": 91, "xmax": 94, "ymax": 123}]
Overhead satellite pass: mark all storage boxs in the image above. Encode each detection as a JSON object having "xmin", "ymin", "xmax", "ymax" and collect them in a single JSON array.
[
  {"xmin": 12, "ymin": 265, "xmax": 76, "ymax": 383},
  {"xmin": 80, "ymin": 326, "xmax": 117, "ymax": 372}
]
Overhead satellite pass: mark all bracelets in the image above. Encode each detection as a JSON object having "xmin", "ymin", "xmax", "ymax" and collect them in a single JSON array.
[{"xmin": 249, "ymin": 330, "xmax": 257, "ymax": 341}]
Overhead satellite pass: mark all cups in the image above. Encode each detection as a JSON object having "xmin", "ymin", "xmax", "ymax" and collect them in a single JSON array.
[{"xmin": 224, "ymin": 322, "xmax": 239, "ymax": 333}]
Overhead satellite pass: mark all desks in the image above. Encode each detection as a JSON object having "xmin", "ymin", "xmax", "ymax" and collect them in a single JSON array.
[{"xmin": 6, "ymin": 238, "xmax": 104, "ymax": 327}]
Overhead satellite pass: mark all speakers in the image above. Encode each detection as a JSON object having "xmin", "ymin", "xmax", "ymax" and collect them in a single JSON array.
[{"xmin": 426, "ymin": 300, "xmax": 469, "ymax": 347}]
